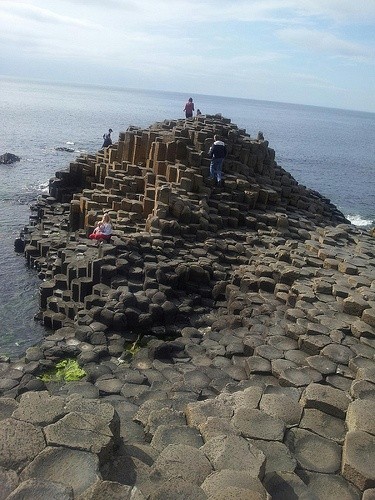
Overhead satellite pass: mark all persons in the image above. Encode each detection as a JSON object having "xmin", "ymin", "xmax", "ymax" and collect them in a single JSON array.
[
  {"xmin": 183, "ymin": 97, "xmax": 194, "ymax": 118},
  {"xmin": 208, "ymin": 134, "xmax": 226, "ymax": 185},
  {"xmin": 103, "ymin": 128, "xmax": 113, "ymax": 147},
  {"xmin": 89, "ymin": 213, "xmax": 112, "ymax": 240}
]
